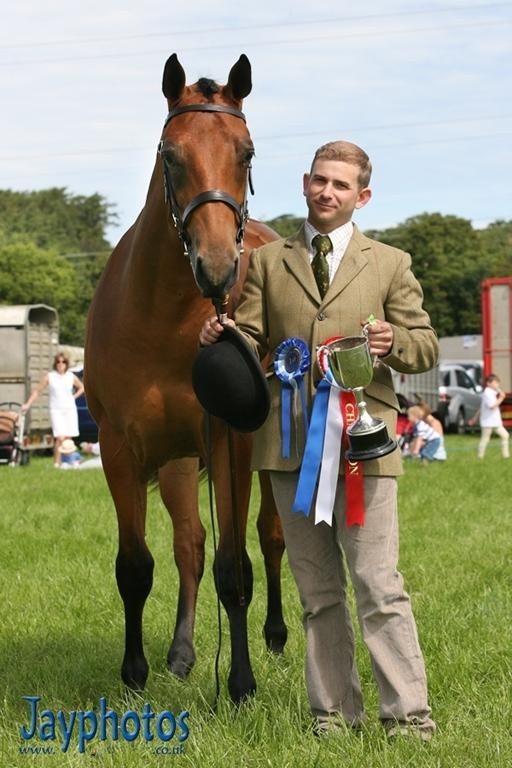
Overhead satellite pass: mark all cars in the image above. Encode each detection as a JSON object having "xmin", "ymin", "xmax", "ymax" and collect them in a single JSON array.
[{"xmin": 437, "ymin": 358, "xmax": 487, "ymax": 433}]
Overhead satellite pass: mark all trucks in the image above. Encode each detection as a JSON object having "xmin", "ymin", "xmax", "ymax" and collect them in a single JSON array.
[
  {"xmin": 481, "ymin": 277, "xmax": 512, "ymax": 437},
  {"xmin": 0, "ymin": 305, "xmax": 58, "ymax": 451}
]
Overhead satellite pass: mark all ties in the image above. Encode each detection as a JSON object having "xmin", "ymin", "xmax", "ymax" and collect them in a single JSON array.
[{"xmin": 311, "ymin": 235, "xmax": 333, "ymax": 300}]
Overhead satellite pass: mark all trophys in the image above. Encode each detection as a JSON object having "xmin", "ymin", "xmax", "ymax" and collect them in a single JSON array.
[{"xmin": 322, "ymin": 321, "xmax": 399, "ymax": 463}]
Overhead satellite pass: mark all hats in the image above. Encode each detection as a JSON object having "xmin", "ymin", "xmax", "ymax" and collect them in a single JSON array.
[
  {"xmin": 192, "ymin": 324, "xmax": 271, "ymax": 433},
  {"xmin": 58, "ymin": 439, "xmax": 76, "ymax": 453}
]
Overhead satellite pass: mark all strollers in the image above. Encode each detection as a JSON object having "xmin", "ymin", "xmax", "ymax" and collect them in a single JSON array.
[{"xmin": 0, "ymin": 402, "xmax": 31, "ymax": 468}]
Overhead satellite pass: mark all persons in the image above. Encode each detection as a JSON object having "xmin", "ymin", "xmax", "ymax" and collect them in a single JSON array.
[
  {"xmin": 21, "ymin": 351, "xmax": 89, "ymax": 467},
  {"xmin": 198, "ymin": 140, "xmax": 443, "ymax": 741},
  {"xmin": 467, "ymin": 374, "xmax": 511, "ymax": 460},
  {"xmin": 80, "ymin": 436, "xmax": 101, "ymax": 455},
  {"xmin": 418, "ymin": 399, "xmax": 447, "ymax": 460},
  {"xmin": 405, "ymin": 405, "xmax": 440, "ymax": 465}
]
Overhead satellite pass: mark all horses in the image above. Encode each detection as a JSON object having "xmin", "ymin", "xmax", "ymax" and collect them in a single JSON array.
[{"xmin": 82, "ymin": 52, "xmax": 289, "ymax": 708}]
